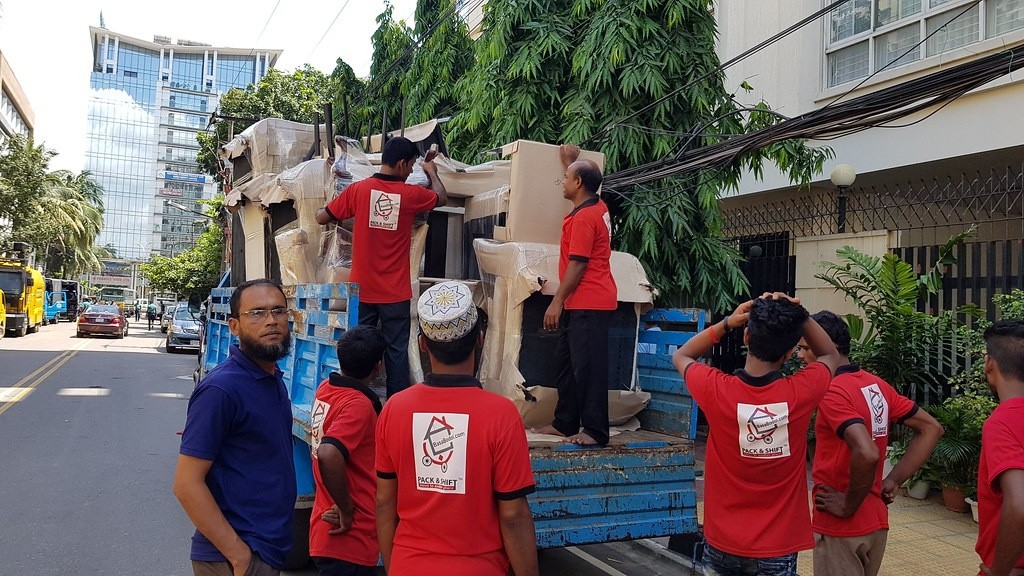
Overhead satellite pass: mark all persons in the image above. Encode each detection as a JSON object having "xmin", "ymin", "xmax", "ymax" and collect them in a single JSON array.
[
  {"xmin": 314, "ymin": 135, "xmax": 449, "ymax": 399},
  {"xmin": 671, "ymin": 292, "xmax": 841, "ymax": 576},
  {"xmin": 158, "ymin": 301, "xmax": 165, "ymax": 325},
  {"xmin": 172, "ymin": 278, "xmax": 297, "ymax": 576},
  {"xmin": 973, "ymin": 318, "xmax": 1024, "ymax": 576},
  {"xmin": 530, "ymin": 143, "xmax": 619, "ymax": 446},
  {"xmin": 84, "ymin": 298, "xmax": 98, "ymax": 308},
  {"xmin": 146, "ymin": 301, "xmax": 157, "ymax": 331},
  {"xmin": 374, "ymin": 279, "xmax": 540, "ymax": 576},
  {"xmin": 105, "ymin": 299, "xmax": 109, "ymax": 305},
  {"xmin": 134, "ymin": 300, "xmax": 141, "ymax": 322},
  {"xmin": 308, "ymin": 324, "xmax": 397, "ymax": 576},
  {"xmin": 798, "ymin": 310, "xmax": 944, "ymax": 576},
  {"xmin": 110, "ymin": 300, "xmax": 116, "ymax": 305}
]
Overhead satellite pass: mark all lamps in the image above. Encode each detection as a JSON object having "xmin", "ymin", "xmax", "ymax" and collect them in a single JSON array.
[{"xmin": 830, "ymin": 165, "xmax": 856, "ymax": 232}]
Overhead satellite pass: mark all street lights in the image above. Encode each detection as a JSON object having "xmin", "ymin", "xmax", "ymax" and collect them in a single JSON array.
[{"xmin": 137, "ymin": 241, "xmax": 153, "ymax": 298}]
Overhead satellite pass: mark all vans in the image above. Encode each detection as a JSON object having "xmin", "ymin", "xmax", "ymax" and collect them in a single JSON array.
[{"xmin": 138, "ymin": 299, "xmax": 148, "ymax": 312}]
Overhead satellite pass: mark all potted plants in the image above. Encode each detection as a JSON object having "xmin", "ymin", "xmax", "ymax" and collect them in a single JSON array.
[{"xmin": 805, "ymin": 222, "xmax": 1024, "ymax": 523}]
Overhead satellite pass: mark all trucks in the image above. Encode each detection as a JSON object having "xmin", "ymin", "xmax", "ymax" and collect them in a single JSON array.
[
  {"xmin": 0, "ymin": 242, "xmax": 137, "ymax": 339},
  {"xmin": 146, "ymin": 292, "xmax": 177, "ymax": 319},
  {"xmin": 188, "ymin": 267, "xmax": 705, "ymax": 567}
]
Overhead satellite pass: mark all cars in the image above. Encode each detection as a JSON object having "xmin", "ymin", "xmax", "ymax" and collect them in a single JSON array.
[
  {"xmin": 76, "ymin": 304, "xmax": 129, "ymax": 339},
  {"xmin": 160, "ymin": 301, "xmax": 202, "ymax": 353}
]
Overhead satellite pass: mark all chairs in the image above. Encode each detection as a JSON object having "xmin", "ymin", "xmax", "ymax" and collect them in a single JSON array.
[{"xmin": 302, "ymin": 94, "xmax": 405, "ymax": 165}]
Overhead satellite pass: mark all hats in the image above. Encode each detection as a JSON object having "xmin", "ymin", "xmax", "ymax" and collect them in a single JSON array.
[{"xmin": 417, "ymin": 281, "xmax": 479, "ymax": 343}]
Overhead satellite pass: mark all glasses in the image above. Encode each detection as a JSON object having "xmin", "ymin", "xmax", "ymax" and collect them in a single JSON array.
[{"xmin": 233, "ymin": 308, "xmax": 293, "ymax": 322}]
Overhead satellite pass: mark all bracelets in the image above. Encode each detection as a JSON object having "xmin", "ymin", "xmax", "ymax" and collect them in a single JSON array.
[
  {"xmin": 708, "ymin": 325, "xmax": 720, "ymax": 344},
  {"xmin": 723, "ymin": 316, "xmax": 733, "ymax": 333}
]
